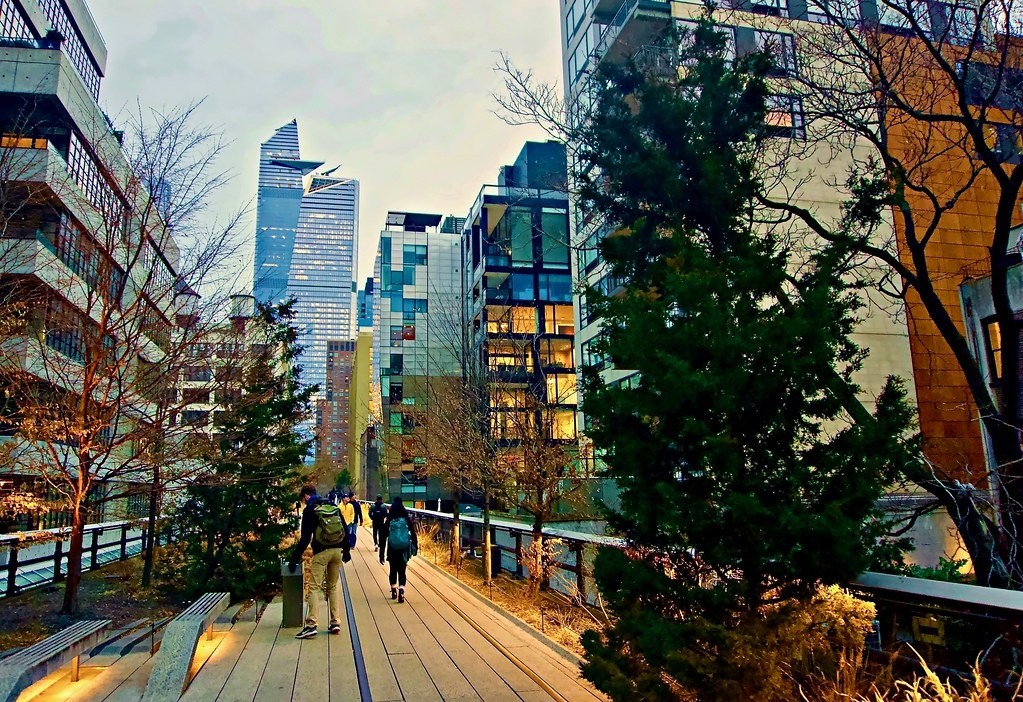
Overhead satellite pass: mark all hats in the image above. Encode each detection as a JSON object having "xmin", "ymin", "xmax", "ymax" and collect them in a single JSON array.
[
  {"xmin": 376, "ymin": 494, "xmax": 382, "ymax": 500},
  {"xmin": 341, "ymin": 493, "xmax": 349, "ymax": 499}
]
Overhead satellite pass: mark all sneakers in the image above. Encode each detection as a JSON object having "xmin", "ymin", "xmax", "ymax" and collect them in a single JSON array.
[
  {"xmin": 327, "ymin": 624, "xmax": 340, "ymax": 632},
  {"xmin": 295, "ymin": 625, "xmax": 318, "ymax": 639}
]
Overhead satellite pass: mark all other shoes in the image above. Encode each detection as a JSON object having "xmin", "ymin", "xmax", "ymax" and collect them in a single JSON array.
[
  {"xmin": 375, "ymin": 545, "xmax": 378, "ymax": 552},
  {"xmin": 398, "ymin": 588, "xmax": 404, "ymax": 603},
  {"xmin": 390, "ymin": 587, "xmax": 397, "ymax": 599}
]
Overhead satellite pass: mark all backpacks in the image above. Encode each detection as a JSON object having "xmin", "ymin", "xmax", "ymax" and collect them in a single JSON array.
[
  {"xmin": 388, "ymin": 514, "xmax": 411, "ymax": 548},
  {"xmin": 314, "ymin": 500, "xmax": 345, "ymax": 545}
]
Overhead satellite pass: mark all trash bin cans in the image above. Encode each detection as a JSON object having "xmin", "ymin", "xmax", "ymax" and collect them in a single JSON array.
[{"xmin": 280, "ymin": 556, "xmax": 306, "ymax": 627}]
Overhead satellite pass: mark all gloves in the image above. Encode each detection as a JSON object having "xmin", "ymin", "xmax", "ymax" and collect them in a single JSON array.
[
  {"xmin": 341, "ymin": 548, "xmax": 352, "ymax": 563},
  {"xmin": 287, "ymin": 557, "xmax": 297, "ymax": 573},
  {"xmin": 380, "ymin": 554, "xmax": 385, "ymax": 565},
  {"xmin": 413, "ymin": 547, "xmax": 418, "ymax": 557}
]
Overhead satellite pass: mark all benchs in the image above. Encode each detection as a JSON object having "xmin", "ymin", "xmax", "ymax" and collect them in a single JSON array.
[
  {"xmin": 143, "ymin": 592, "xmax": 230, "ymax": 702},
  {"xmin": 0, "ymin": 619, "xmax": 112, "ymax": 702}
]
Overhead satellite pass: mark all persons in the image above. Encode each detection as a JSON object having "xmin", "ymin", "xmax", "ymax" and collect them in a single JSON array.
[
  {"xmin": 327, "ymin": 491, "xmax": 343, "ymax": 503},
  {"xmin": 338, "ymin": 493, "xmax": 355, "ymax": 536},
  {"xmin": 380, "ymin": 497, "xmax": 418, "ymax": 603},
  {"xmin": 369, "ymin": 494, "xmax": 387, "ymax": 551},
  {"xmin": 288, "ymin": 485, "xmax": 351, "ymax": 639},
  {"xmin": 348, "ymin": 492, "xmax": 363, "ymax": 550}
]
